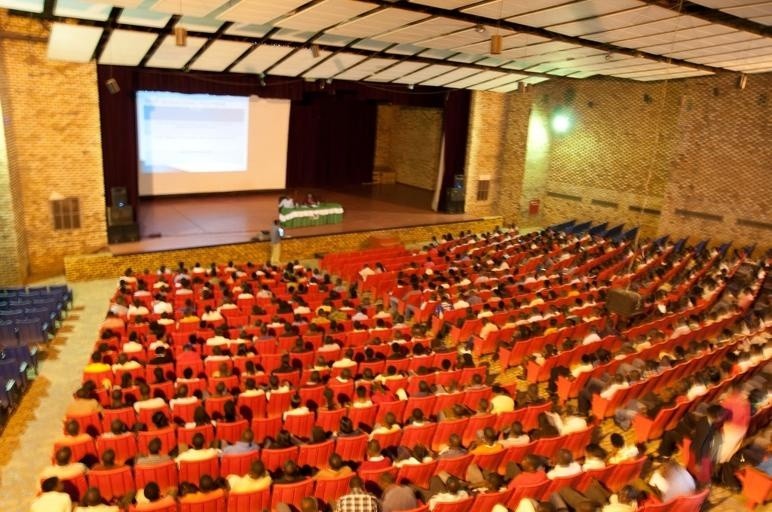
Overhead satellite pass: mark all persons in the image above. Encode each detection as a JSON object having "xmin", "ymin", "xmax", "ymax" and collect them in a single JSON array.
[
  {"xmin": 31, "ymin": 220, "xmax": 772, "ymax": 512},
  {"xmin": 278, "ymin": 195, "xmax": 295, "ymax": 209},
  {"xmin": 304, "ymin": 193, "xmax": 320, "ymax": 208}
]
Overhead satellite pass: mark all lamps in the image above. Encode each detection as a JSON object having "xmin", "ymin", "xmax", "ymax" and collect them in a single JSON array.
[
  {"xmin": 734, "ymin": 30, "xmax": 758, "ymax": 89},
  {"xmin": 490, "ymin": 1, "xmax": 502, "ymax": 55},
  {"xmin": 310, "ymin": 2, "xmax": 321, "ymax": 59},
  {"xmin": 104, "ymin": 0, "xmax": 187, "ymax": 95}
]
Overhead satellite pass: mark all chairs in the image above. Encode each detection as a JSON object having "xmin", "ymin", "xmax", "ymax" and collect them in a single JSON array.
[
  {"xmin": 31, "ymin": 217, "xmax": 771, "ymax": 512},
  {"xmin": 0, "ymin": 285, "xmax": 73, "ymax": 439},
  {"xmin": 2, "ymin": 220, "xmax": 772, "ymax": 511}
]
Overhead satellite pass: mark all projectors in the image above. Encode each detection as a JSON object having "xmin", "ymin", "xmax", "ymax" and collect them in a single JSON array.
[{"xmin": 607, "ymin": 287, "xmax": 646, "ymax": 318}]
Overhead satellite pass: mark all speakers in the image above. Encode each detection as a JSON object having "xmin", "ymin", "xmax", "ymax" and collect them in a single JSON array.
[
  {"xmin": 735, "ymin": 73, "xmax": 748, "ymax": 90},
  {"xmin": 106, "ymin": 77, "xmax": 120, "ymax": 96},
  {"xmin": 309, "ymin": 44, "xmax": 320, "ymax": 58},
  {"xmin": 491, "ymin": 35, "xmax": 500, "ymax": 56},
  {"xmin": 445, "ymin": 174, "xmax": 466, "ymax": 214},
  {"xmin": 517, "ymin": 80, "xmax": 526, "ymax": 93},
  {"xmin": 107, "ymin": 185, "xmax": 141, "ymax": 244},
  {"xmin": 174, "ymin": 25, "xmax": 186, "ymax": 48}
]
